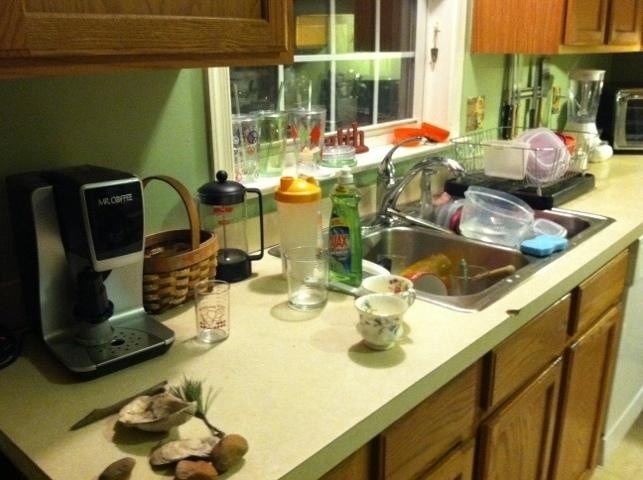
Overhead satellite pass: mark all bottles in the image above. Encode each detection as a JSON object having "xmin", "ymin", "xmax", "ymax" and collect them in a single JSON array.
[{"xmin": 197, "ymin": 172, "xmax": 263, "ymax": 282}]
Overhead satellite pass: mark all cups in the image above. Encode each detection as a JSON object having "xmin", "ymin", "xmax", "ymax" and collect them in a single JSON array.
[
  {"xmin": 192, "ymin": 276, "xmax": 231, "ymax": 344},
  {"xmin": 449, "ymin": 260, "xmax": 487, "ymax": 294},
  {"xmin": 351, "ymin": 292, "xmax": 406, "ymax": 351},
  {"xmin": 228, "ymin": 102, "xmax": 326, "ymax": 183},
  {"xmin": 282, "ymin": 244, "xmax": 330, "ymax": 310},
  {"xmin": 359, "ymin": 273, "xmax": 417, "ymax": 313},
  {"xmin": 272, "ymin": 175, "xmax": 323, "ymax": 283}
]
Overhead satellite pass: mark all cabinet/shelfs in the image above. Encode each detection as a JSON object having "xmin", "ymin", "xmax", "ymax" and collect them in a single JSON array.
[
  {"xmin": 550, "ymin": 242, "xmax": 637, "ymax": 480},
  {"xmin": 0, "ymin": 0, "xmax": 298, "ymax": 83},
  {"xmin": 382, "ymin": 357, "xmax": 485, "ymax": 480},
  {"xmin": 316, "ymin": 428, "xmax": 382, "ymax": 479},
  {"xmin": 486, "ymin": 287, "xmax": 571, "ymax": 480},
  {"xmin": 469, "ymin": 0, "xmax": 641, "ymax": 57}
]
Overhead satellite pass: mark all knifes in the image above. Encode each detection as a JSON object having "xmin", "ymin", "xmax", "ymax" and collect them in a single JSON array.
[
  {"xmin": 529, "ymin": 56, "xmax": 541, "ymax": 129},
  {"xmin": 68, "ymin": 380, "xmax": 167, "ymax": 432},
  {"xmin": 502, "ymin": 53, "xmax": 515, "ymax": 140}
]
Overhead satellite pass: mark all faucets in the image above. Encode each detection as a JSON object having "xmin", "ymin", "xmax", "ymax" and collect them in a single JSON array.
[{"xmin": 376, "ymin": 135, "xmax": 466, "ymax": 224}]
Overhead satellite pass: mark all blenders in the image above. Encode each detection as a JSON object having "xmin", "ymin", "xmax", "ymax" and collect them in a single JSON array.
[
  {"xmin": 334, "ymin": 67, "xmax": 368, "ymax": 127},
  {"xmin": 564, "ymin": 69, "xmax": 613, "ymax": 165}
]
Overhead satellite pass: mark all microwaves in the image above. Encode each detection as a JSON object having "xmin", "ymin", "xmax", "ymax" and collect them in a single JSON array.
[{"xmin": 603, "ymin": 84, "xmax": 642, "ymax": 151}]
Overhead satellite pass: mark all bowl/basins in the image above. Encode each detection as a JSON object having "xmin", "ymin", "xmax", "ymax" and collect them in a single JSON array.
[
  {"xmin": 421, "ymin": 124, "xmax": 448, "ymax": 142},
  {"xmin": 393, "ymin": 127, "xmax": 424, "ymax": 148},
  {"xmin": 321, "ymin": 144, "xmax": 358, "ymax": 167},
  {"xmin": 409, "ymin": 273, "xmax": 449, "ymax": 297},
  {"xmin": 455, "ymin": 185, "xmax": 533, "ymax": 252}
]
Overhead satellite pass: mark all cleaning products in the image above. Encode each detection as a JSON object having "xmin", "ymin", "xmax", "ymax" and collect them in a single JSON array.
[{"xmin": 328, "ymin": 166, "xmax": 364, "ymax": 288}]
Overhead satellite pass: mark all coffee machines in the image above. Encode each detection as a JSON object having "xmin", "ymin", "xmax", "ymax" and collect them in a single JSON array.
[{"xmin": 1, "ymin": 164, "xmax": 176, "ymax": 378}]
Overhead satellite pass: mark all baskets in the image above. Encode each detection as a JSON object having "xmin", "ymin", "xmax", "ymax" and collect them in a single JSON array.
[
  {"xmin": 449, "ymin": 125, "xmax": 600, "ymax": 189},
  {"xmin": 142, "ymin": 175, "xmax": 219, "ymax": 315}
]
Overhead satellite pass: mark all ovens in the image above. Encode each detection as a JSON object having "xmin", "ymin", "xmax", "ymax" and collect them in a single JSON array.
[{"xmin": 598, "ymin": 237, "xmax": 642, "ymax": 468}]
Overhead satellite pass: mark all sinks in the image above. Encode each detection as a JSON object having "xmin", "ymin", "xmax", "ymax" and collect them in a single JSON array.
[
  {"xmin": 422, "ymin": 199, "xmax": 591, "ymax": 249},
  {"xmin": 361, "ymin": 228, "xmax": 530, "ymax": 296}
]
